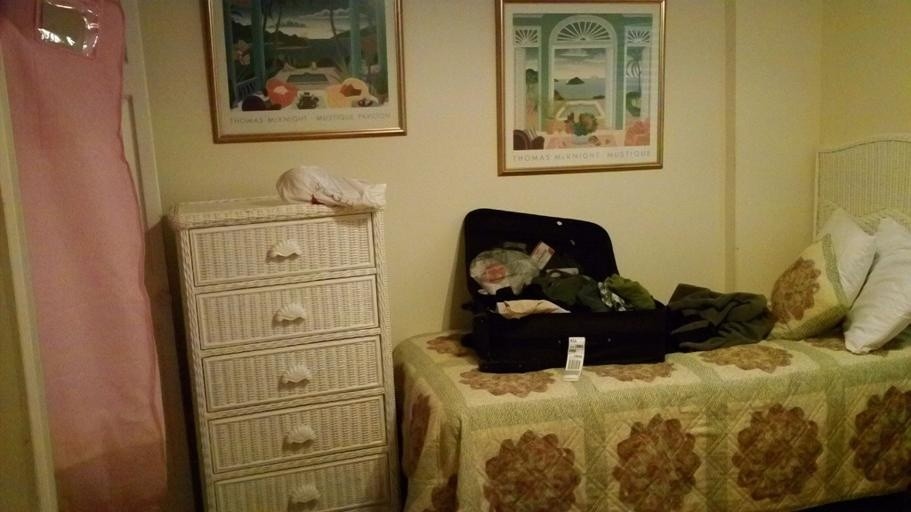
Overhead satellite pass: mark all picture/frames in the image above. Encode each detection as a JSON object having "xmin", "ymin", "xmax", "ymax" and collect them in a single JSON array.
[
  {"xmin": 200, "ymin": 0, "xmax": 410, "ymax": 142},
  {"xmin": 494, "ymin": 0, "xmax": 668, "ymax": 175}
]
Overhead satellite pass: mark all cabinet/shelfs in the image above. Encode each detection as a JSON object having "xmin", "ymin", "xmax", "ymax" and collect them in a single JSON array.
[{"xmin": 163, "ymin": 182, "xmax": 404, "ymax": 511}]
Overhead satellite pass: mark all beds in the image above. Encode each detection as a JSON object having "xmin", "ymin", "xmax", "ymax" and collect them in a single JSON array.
[{"xmin": 391, "ymin": 129, "xmax": 911, "ymax": 511}]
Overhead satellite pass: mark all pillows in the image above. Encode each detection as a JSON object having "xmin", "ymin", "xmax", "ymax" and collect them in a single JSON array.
[
  {"xmin": 764, "ymin": 232, "xmax": 849, "ymax": 341},
  {"xmin": 838, "ymin": 215, "xmax": 911, "ymax": 354},
  {"xmin": 818, "ymin": 203, "xmax": 878, "ymax": 308}
]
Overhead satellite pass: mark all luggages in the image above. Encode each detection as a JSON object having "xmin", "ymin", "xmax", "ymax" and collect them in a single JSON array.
[{"xmin": 463, "ymin": 209, "xmax": 671, "ymax": 373}]
